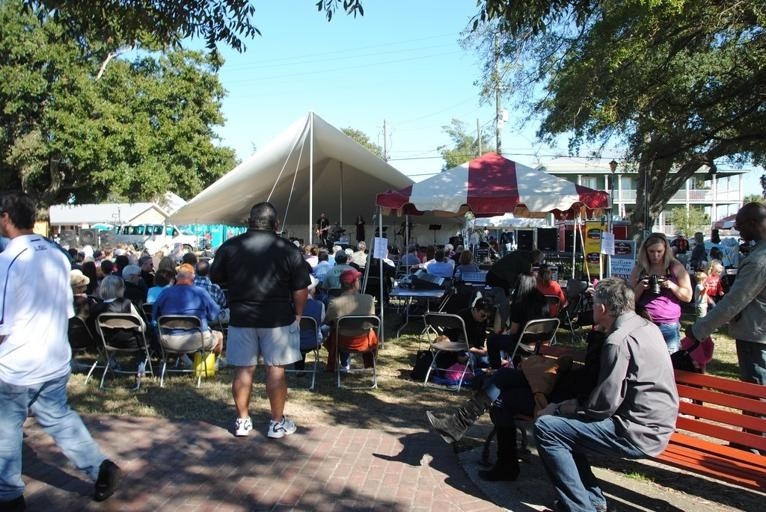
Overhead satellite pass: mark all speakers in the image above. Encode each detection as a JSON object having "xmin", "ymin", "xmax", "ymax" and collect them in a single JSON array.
[
  {"xmin": 80, "ymin": 229, "xmax": 99, "ymax": 248},
  {"xmin": 537, "ymin": 227, "xmax": 560, "ymax": 256},
  {"xmin": 99, "ymin": 231, "xmax": 117, "ymax": 250},
  {"xmin": 60, "ymin": 230, "xmax": 76, "ymax": 247},
  {"xmin": 449, "ymin": 238, "xmax": 463, "ymax": 250},
  {"xmin": 565, "ymin": 229, "xmax": 581, "ymax": 253},
  {"xmin": 517, "ymin": 229, "xmax": 533, "ymax": 253}
]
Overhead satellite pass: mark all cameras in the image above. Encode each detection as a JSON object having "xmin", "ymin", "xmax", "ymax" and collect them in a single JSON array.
[{"xmin": 644, "ymin": 274, "xmax": 664, "ymax": 295}]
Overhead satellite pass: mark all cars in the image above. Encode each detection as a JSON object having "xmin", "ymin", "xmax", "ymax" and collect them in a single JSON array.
[{"xmin": 703, "ymin": 237, "xmax": 741, "ymax": 284}]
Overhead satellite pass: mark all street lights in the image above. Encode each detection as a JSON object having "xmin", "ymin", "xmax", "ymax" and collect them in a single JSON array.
[{"xmin": 607, "ymin": 157, "xmax": 618, "ymax": 223}]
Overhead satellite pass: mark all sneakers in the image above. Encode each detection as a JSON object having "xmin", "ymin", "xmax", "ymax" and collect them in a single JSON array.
[
  {"xmin": 295, "ymin": 368, "xmax": 306, "ymax": 376},
  {"xmin": 232, "ymin": 414, "xmax": 253, "ymax": 436},
  {"xmin": 0, "ymin": 495, "xmax": 25, "ymax": 511},
  {"xmin": 340, "ymin": 362, "xmax": 350, "ymax": 373},
  {"xmin": 92, "ymin": 456, "xmax": 121, "ymax": 501},
  {"xmin": 268, "ymin": 417, "xmax": 298, "ymax": 438}
]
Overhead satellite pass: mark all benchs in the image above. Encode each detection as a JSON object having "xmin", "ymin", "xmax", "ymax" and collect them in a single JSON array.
[{"xmin": 474, "ymin": 340, "xmax": 765, "ymax": 512}]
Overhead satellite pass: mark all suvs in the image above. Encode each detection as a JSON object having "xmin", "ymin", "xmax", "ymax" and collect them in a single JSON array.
[{"xmin": 113, "ymin": 222, "xmax": 208, "ymax": 254}]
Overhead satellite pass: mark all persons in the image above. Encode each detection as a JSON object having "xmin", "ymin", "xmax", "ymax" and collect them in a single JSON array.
[
  {"xmin": 533, "ymin": 277, "xmax": 682, "ymax": 511},
  {"xmin": 207, "ymin": 202, "xmax": 310, "ymax": 440},
  {"xmin": 316, "ymin": 211, "xmax": 329, "ymax": 245},
  {"xmin": 424, "ymin": 301, "xmax": 655, "ymax": 483},
  {"xmin": 45, "ymin": 228, "xmax": 748, "ymax": 390},
  {"xmin": 353, "ymin": 215, "xmax": 366, "ymax": 242},
  {"xmin": 0, "ymin": 192, "xmax": 120, "ymax": 511},
  {"xmin": 685, "ymin": 202, "xmax": 766, "ymax": 456}
]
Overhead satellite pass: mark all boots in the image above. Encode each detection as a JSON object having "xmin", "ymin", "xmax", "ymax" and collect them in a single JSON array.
[
  {"xmin": 425, "ymin": 386, "xmax": 495, "ymax": 444},
  {"xmin": 479, "ymin": 418, "xmax": 526, "ymax": 481}
]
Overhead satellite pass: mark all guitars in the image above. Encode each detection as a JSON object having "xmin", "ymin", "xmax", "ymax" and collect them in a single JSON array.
[{"xmin": 315, "ymin": 226, "xmax": 331, "ymax": 237}]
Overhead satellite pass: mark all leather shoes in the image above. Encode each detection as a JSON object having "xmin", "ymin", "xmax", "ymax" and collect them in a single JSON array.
[{"xmin": 552, "ymin": 496, "xmax": 610, "ymax": 511}]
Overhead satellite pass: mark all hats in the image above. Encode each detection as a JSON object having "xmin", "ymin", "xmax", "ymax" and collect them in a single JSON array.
[
  {"xmin": 339, "ymin": 268, "xmax": 362, "ymax": 284},
  {"xmin": 175, "ymin": 262, "xmax": 195, "ymax": 274},
  {"xmin": 69, "ymin": 268, "xmax": 90, "ymax": 286},
  {"xmin": 334, "ymin": 249, "xmax": 347, "ymax": 260}
]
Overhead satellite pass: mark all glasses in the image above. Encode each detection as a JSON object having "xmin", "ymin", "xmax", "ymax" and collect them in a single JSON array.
[
  {"xmin": 733, "ymin": 217, "xmax": 759, "ymax": 232},
  {"xmin": 477, "ymin": 310, "xmax": 490, "ymax": 318}
]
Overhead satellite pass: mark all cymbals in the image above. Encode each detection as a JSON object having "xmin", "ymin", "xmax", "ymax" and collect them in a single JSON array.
[{"xmin": 336, "ymin": 229, "xmax": 348, "ymax": 233}]
